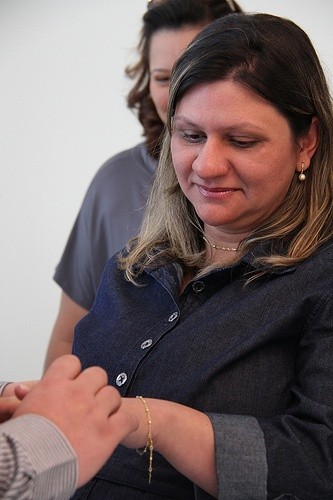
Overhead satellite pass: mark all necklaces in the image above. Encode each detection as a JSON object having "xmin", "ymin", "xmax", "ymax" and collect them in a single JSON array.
[{"xmin": 201, "ymin": 236, "xmax": 241, "ymax": 253}]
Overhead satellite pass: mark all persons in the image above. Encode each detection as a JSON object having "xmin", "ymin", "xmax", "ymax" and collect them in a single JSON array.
[
  {"xmin": 0, "ymin": 13, "xmax": 333, "ymax": 500},
  {"xmin": 40, "ymin": 0, "xmax": 245, "ymax": 380},
  {"xmin": 0, "ymin": 355, "xmax": 140, "ymax": 500}
]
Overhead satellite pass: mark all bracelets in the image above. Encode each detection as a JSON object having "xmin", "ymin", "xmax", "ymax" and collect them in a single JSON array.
[{"xmin": 134, "ymin": 396, "xmax": 153, "ymax": 488}]
